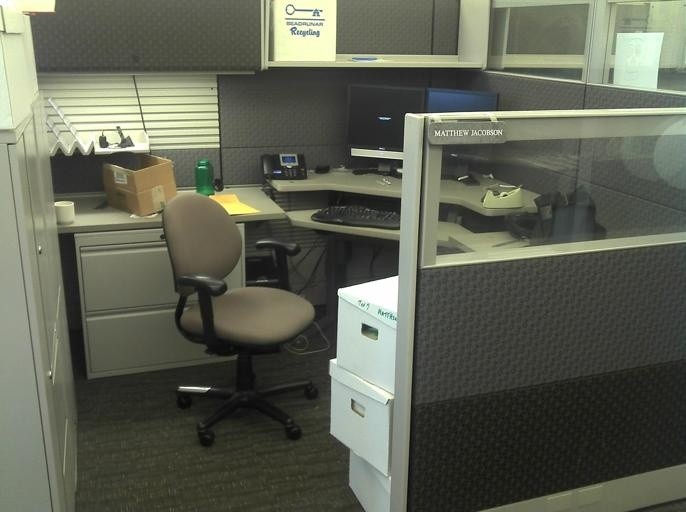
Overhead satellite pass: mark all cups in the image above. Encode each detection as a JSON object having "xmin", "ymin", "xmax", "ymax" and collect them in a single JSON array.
[
  {"xmin": 54, "ymin": 201, "xmax": 75, "ymax": 225},
  {"xmin": 195, "ymin": 158, "xmax": 214, "ymax": 197}
]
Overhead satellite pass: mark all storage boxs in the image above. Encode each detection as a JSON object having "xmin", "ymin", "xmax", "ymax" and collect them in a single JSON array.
[
  {"xmin": 327, "ymin": 357, "xmax": 394, "ymax": 479},
  {"xmin": 335, "ymin": 275, "xmax": 398, "ymax": 394},
  {"xmin": 0, "ymin": 3, "xmax": 40, "ymax": 131},
  {"xmin": 102, "ymin": 154, "xmax": 177, "ymax": 218},
  {"xmin": 348, "ymin": 449, "xmax": 392, "ymax": 512}
]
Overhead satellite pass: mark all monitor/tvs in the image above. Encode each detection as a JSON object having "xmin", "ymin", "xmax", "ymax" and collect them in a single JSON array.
[
  {"xmin": 345, "ymin": 82, "xmax": 424, "ymax": 178},
  {"xmin": 426, "ymin": 86, "xmax": 498, "ymax": 187}
]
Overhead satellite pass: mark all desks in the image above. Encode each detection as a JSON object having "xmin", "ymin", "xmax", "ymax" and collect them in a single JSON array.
[
  {"xmin": 267, "ymin": 165, "xmax": 543, "ymax": 251},
  {"xmin": 53, "ymin": 182, "xmax": 288, "ymax": 236}
]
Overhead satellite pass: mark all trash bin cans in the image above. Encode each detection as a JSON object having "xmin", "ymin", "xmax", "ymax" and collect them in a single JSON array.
[{"xmin": 246, "ymin": 245, "xmax": 283, "ymax": 349}]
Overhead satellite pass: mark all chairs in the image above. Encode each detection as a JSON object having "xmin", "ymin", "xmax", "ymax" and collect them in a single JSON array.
[{"xmin": 162, "ymin": 192, "xmax": 315, "ymax": 446}]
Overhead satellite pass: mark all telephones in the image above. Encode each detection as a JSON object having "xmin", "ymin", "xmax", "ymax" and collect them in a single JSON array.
[{"xmin": 262, "ymin": 153, "xmax": 306, "ymax": 180}]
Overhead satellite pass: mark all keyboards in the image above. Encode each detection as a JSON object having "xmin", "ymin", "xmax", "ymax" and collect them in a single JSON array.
[{"xmin": 311, "ymin": 204, "xmax": 402, "ymax": 230}]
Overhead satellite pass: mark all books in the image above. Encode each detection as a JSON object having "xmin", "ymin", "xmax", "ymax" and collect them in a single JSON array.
[{"xmin": 268, "ymin": 1, "xmax": 337, "ymax": 62}]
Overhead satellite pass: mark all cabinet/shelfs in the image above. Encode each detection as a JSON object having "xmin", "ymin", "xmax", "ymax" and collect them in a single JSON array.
[{"xmin": 72, "ymin": 222, "xmax": 246, "ymax": 381}]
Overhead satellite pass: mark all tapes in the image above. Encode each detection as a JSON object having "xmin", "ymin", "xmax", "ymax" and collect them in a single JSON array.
[{"xmin": 500, "ymin": 192, "xmax": 508, "ymax": 197}]
